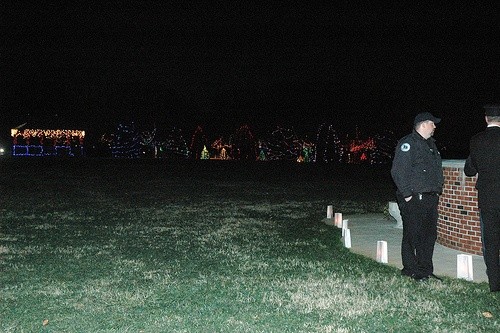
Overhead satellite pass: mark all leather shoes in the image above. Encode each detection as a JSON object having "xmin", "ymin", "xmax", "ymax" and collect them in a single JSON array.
[
  {"xmin": 414, "ymin": 274, "xmax": 443, "ymax": 281},
  {"xmin": 400, "ymin": 268, "xmax": 414, "ymax": 275}
]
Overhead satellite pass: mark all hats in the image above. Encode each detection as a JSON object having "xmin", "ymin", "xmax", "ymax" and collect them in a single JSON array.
[
  {"xmin": 414, "ymin": 112, "xmax": 441, "ymax": 125},
  {"xmin": 483, "ymin": 103, "xmax": 500, "ymax": 117}
]
